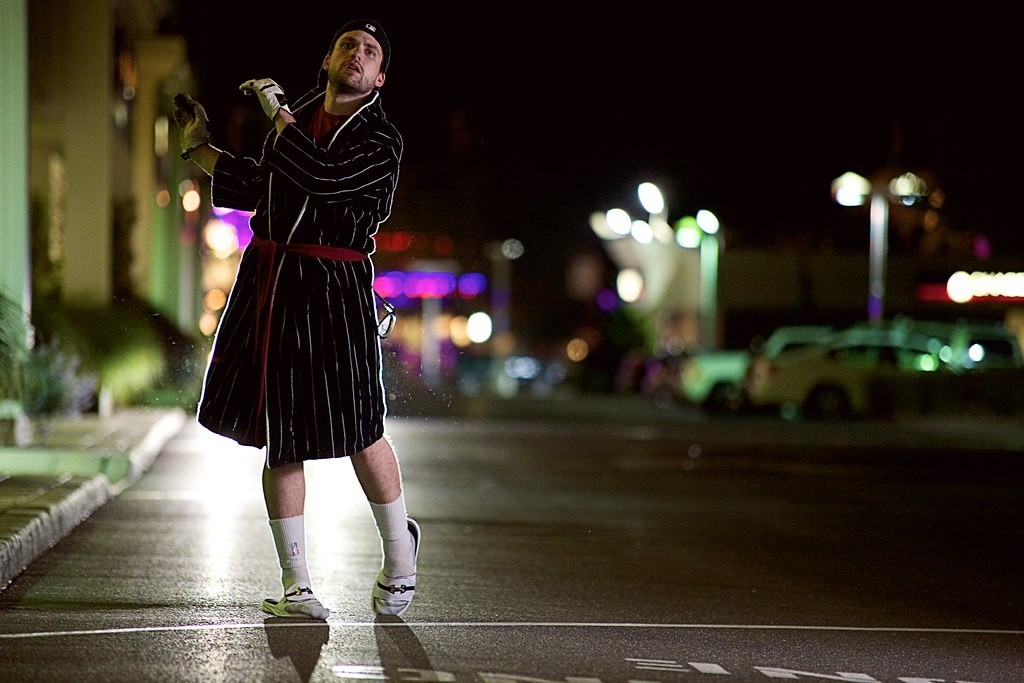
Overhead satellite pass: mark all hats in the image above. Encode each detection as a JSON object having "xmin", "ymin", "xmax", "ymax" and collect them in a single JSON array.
[{"xmin": 328, "ymin": 17, "xmax": 392, "ymax": 72}]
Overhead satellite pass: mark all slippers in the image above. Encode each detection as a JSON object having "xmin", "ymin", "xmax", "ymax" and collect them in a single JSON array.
[
  {"xmin": 260, "ymin": 581, "xmax": 323, "ymax": 618},
  {"xmin": 370, "ymin": 515, "xmax": 422, "ymax": 618}
]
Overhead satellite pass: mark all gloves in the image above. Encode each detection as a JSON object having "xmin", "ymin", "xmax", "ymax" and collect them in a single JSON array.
[
  {"xmin": 240, "ymin": 78, "xmax": 295, "ymax": 122},
  {"xmin": 170, "ymin": 90, "xmax": 209, "ymax": 162}
]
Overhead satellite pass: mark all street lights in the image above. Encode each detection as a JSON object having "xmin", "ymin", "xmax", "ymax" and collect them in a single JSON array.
[{"xmin": 836, "ymin": 171, "xmax": 924, "ymax": 326}]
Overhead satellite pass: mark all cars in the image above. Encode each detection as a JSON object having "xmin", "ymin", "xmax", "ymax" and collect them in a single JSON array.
[
  {"xmin": 678, "ymin": 322, "xmax": 868, "ymax": 423},
  {"xmin": 749, "ymin": 326, "xmax": 1005, "ymax": 424}
]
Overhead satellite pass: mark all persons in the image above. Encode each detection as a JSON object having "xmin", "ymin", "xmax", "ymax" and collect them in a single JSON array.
[{"xmin": 176, "ymin": 18, "xmax": 422, "ymax": 618}]
